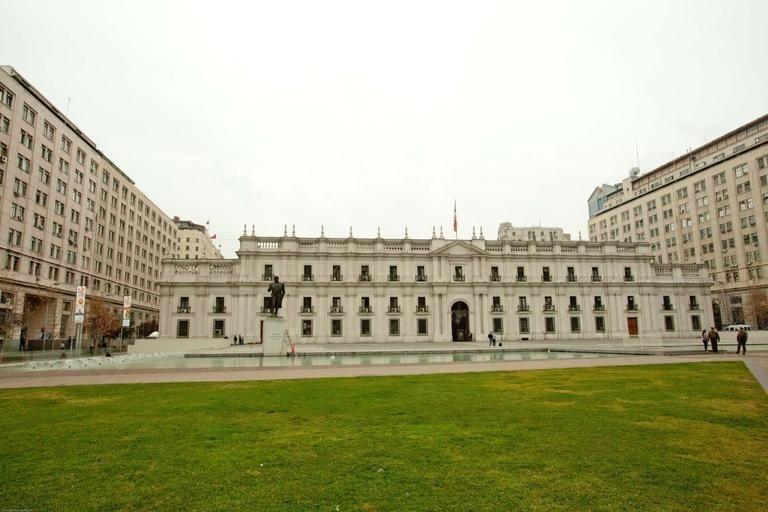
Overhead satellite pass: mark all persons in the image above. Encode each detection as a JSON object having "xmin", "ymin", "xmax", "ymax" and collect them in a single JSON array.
[
  {"xmin": 18, "ymin": 328, "xmax": 27, "ymax": 352},
  {"xmin": 707, "ymin": 326, "xmax": 721, "ymax": 352},
  {"xmin": 735, "ymin": 326, "xmax": 748, "ymax": 355},
  {"xmin": 234, "ymin": 335, "xmax": 237, "ymax": 346},
  {"xmin": 701, "ymin": 328, "xmax": 711, "ymax": 352},
  {"xmin": 238, "ymin": 334, "xmax": 242, "ymax": 344},
  {"xmin": 266, "ymin": 274, "xmax": 286, "ymax": 318},
  {"xmin": 488, "ymin": 331, "xmax": 493, "ymax": 346},
  {"xmin": 492, "ymin": 332, "xmax": 496, "ymax": 347}
]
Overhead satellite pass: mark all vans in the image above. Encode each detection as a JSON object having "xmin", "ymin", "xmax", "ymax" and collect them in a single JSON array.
[{"xmin": 726, "ymin": 325, "xmax": 752, "ymax": 331}]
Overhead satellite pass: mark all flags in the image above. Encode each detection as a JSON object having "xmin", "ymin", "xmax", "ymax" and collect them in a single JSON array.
[{"xmin": 453, "ymin": 198, "xmax": 458, "ymax": 233}]
